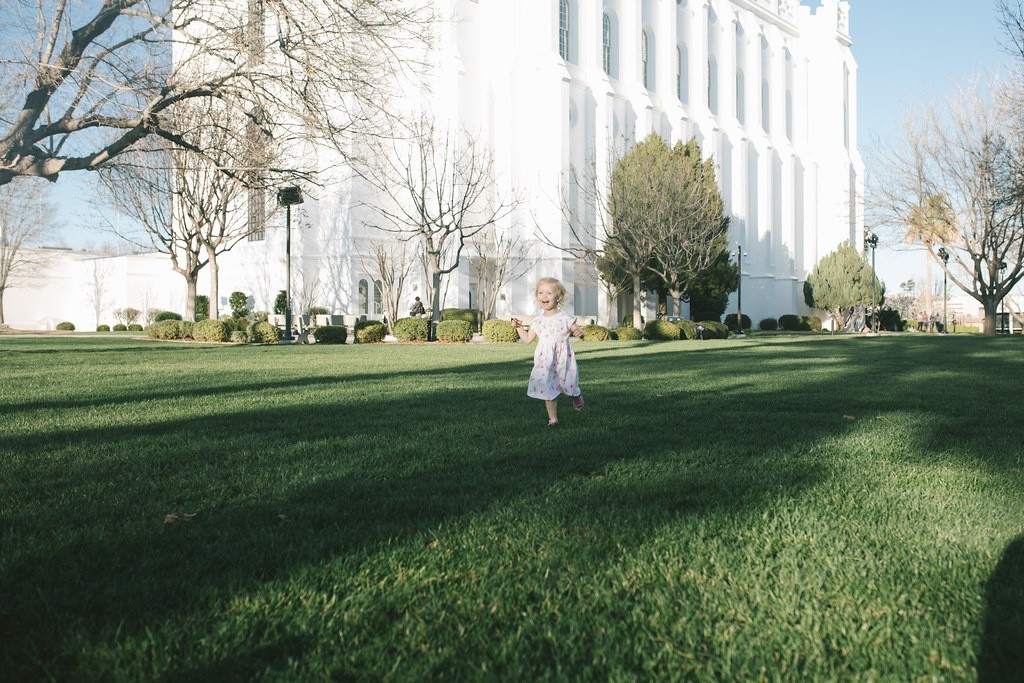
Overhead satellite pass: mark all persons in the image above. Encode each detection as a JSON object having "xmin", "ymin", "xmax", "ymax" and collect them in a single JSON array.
[
  {"xmin": 411, "ymin": 297, "xmax": 423, "ymax": 312},
  {"xmin": 917, "ymin": 311, "xmax": 944, "ymax": 333},
  {"xmin": 511, "ymin": 277, "xmax": 585, "ymax": 427}
]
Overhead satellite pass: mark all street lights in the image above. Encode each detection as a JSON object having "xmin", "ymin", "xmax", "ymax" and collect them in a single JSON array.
[
  {"xmin": 276, "ymin": 185, "xmax": 306, "ymax": 338},
  {"xmin": 937, "ymin": 247, "xmax": 950, "ymax": 334},
  {"xmin": 864, "ymin": 230, "xmax": 879, "ymax": 334},
  {"xmin": 997, "ymin": 261, "xmax": 1007, "ymax": 332},
  {"xmin": 731, "ymin": 244, "xmax": 748, "ymax": 334}
]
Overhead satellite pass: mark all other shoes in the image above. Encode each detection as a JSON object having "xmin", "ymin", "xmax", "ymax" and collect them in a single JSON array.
[
  {"xmin": 548, "ymin": 419, "xmax": 558, "ymax": 426},
  {"xmin": 573, "ymin": 394, "xmax": 584, "ymax": 411}
]
